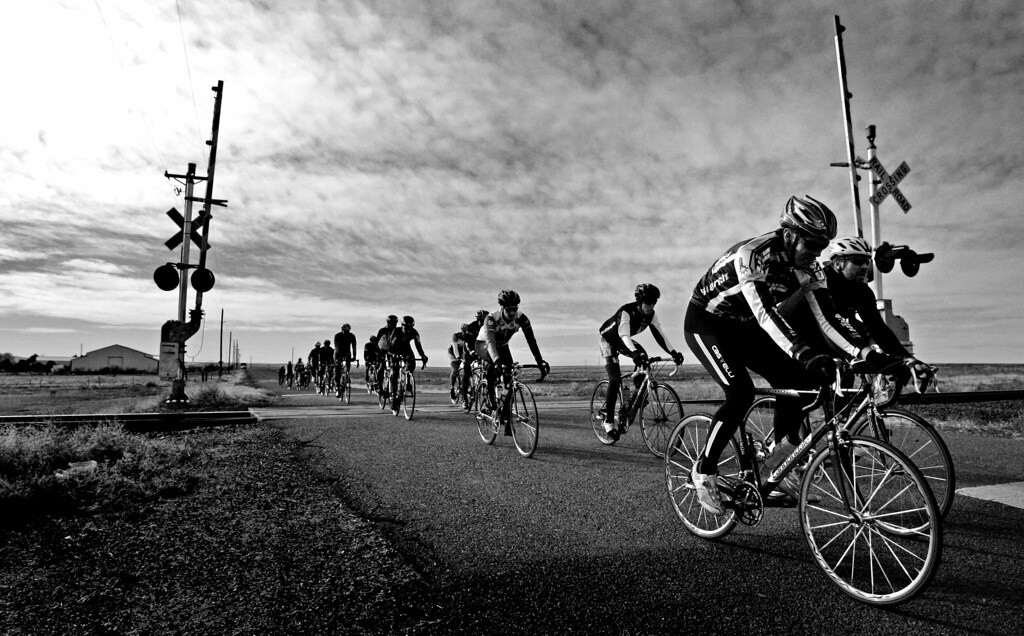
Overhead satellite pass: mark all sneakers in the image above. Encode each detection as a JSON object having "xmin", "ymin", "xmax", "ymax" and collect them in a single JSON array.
[
  {"xmin": 603, "ymin": 418, "xmax": 618, "ymax": 437},
  {"xmin": 690, "ymin": 459, "xmax": 727, "ymax": 516},
  {"xmin": 768, "ymin": 442, "xmax": 819, "ymax": 471}
]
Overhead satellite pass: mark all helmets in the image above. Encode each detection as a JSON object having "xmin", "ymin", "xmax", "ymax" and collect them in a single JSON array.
[
  {"xmin": 286, "ymin": 315, "xmax": 415, "ymax": 364},
  {"xmin": 497, "ymin": 289, "xmax": 520, "ymax": 305},
  {"xmin": 781, "ymin": 194, "xmax": 838, "ymax": 248},
  {"xmin": 634, "ymin": 283, "xmax": 661, "ymax": 298},
  {"xmin": 475, "ymin": 309, "xmax": 490, "ymax": 318},
  {"xmin": 828, "ymin": 237, "xmax": 872, "ymax": 259},
  {"xmin": 461, "ymin": 323, "xmax": 469, "ymax": 330}
]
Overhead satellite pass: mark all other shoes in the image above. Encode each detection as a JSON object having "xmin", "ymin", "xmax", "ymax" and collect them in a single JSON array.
[
  {"xmin": 450, "ymin": 388, "xmax": 456, "ymax": 399},
  {"xmin": 461, "ymin": 394, "xmax": 468, "ymax": 406},
  {"xmin": 286, "ymin": 374, "xmax": 412, "ymax": 410},
  {"xmin": 504, "ymin": 421, "xmax": 516, "ymax": 436}
]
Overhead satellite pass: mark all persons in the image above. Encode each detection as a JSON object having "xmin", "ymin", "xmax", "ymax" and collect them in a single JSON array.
[
  {"xmin": 769, "ymin": 236, "xmax": 934, "ymax": 469},
  {"xmin": 598, "ymin": 283, "xmax": 684, "ymax": 434},
  {"xmin": 333, "ymin": 323, "xmax": 357, "ymax": 397},
  {"xmin": 447, "ymin": 310, "xmax": 489, "ymax": 409},
  {"xmin": 308, "ymin": 340, "xmax": 334, "ymax": 390},
  {"xmin": 475, "ymin": 289, "xmax": 549, "ymax": 437},
  {"xmin": 683, "ymin": 195, "xmax": 890, "ymax": 515},
  {"xmin": 278, "ymin": 358, "xmax": 312, "ymax": 389},
  {"xmin": 364, "ymin": 315, "xmax": 428, "ymax": 410}
]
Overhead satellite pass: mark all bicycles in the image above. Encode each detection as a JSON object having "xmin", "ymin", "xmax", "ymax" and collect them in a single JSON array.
[
  {"xmin": 473, "ymin": 362, "xmax": 550, "ymax": 458},
  {"xmin": 739, "ymin": 350, "xmax": 958, "ymax": 537},
  {"xmin": 663, "ymin": 350, "xmax": 944, "ymax": 606},
  {"xmin": 588, "ymin": 356, "xmax": 685, "ymax": 461},
  {"xmin": 295, "ymin": 357, "xmax": 489, "ymax": 422}
]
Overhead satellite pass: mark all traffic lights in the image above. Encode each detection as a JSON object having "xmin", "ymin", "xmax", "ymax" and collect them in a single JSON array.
[
  {"xmin": 876, "ymin": 244, "xmax": 910, "ymax": 274},
  {"xmin": 899, "ymin": 246, "xmax": 936, "ymax": 276}
]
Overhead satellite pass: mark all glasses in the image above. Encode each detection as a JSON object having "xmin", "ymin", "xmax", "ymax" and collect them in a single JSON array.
[
  {"xmin": 642, "ymin": 299, "xmax": 658, "ymax": 306},
  {"xmin": 505, "ymin": 306, "xmax": 519, "ymax": 313},
  {"xmin": 800, "ymin": 236, "xmax": 830, "ymax": 250},
  {"xmin": 848, "ymin": 255, "xmax": 871, "ymax": 266}
]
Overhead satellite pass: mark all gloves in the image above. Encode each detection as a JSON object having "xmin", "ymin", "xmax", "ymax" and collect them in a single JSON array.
[
  {"xmin": 673, "ymin": 352, "xmax": 685, "ymax": 365},
  {"xmin": 804, "ymin": 354, "xmax": 838, "ymax": 384},
  {"xmin": 632, "ymin": 353, "xmax": 646, "ymax": 367},
  {"xmin": 860, "ymin": 346, "xmax": 900, "ymax": 375}
]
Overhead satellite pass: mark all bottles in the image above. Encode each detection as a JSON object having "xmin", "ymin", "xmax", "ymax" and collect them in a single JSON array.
[
  {"xmin": 495, "ymin": 386, "xmax": 503, "ymax": 398},
  {"xmin": 622, "ymin": 386, "xmax": 630, "ymax": 403},
  {"xmin": 502, "ymin": 384, "xmax": 509, "ymax": 401},
  {"xmin": 822, "ymin": 413, "xmax": 847, "ymax": 442},
  {"xmin": 629, "ymin": 384, "xmax": 640, "ymax": 396},
  {"xmin": 764, "ymin": 433, "xmax": 801, "ymax": 469}
]
